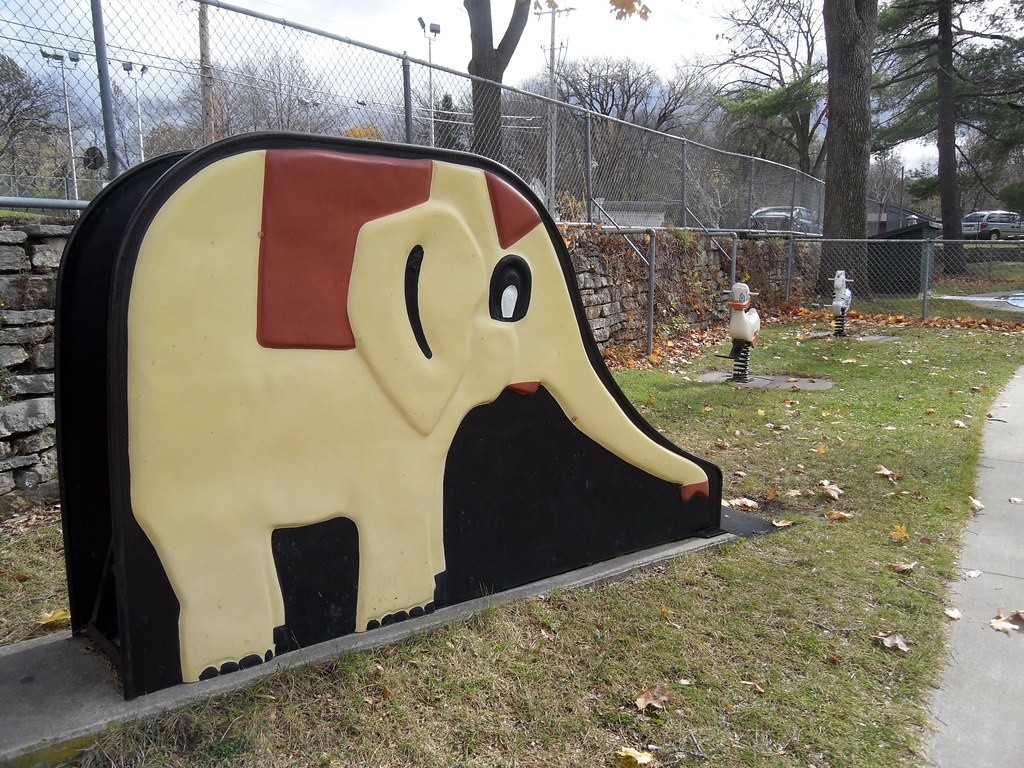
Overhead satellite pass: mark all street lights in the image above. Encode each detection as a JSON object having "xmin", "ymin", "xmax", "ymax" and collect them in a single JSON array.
[
  {"xmin": 123, "ymin": 62, "xmax": 148, "ymax": 163},
  {"xmin": 38, "ymin": 47, "xmax": 81, "ymax": 220},
  {"xmin": 418, "ymin": 16, "xmax": 441, "ymax": 147},
  {"xmin": 296, "ymin": 95, "xmax": 320, "ymax": 133}
]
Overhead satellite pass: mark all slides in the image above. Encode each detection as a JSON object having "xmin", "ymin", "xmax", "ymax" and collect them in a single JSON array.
[{"xmin": 54, "ymin": 130, "xmax": 722, "ymax": 704}]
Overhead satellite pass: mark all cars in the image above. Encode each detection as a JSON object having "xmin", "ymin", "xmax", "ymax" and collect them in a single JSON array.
[{"xmin": 741, "ymin": 206, "xmax": 824, "ymax": 234}]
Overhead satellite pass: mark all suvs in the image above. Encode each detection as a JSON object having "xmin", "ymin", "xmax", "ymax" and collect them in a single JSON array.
[{"xmin": 961, "ymin": 211, "xmax": 1024, "ymax": 239}]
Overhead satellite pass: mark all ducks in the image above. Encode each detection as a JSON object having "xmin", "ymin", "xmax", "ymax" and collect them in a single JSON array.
[{"xmin": 727, "ymin": 282, "xmax": 760, "ymax": 348}]
